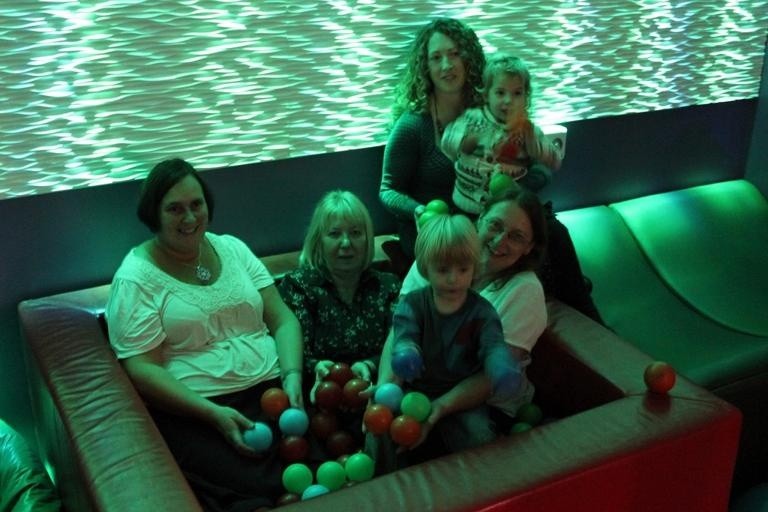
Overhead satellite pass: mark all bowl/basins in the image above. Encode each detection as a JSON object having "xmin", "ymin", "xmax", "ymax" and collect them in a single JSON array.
[
  {"xmin": 154, "ymin": 241, "xmax": 213, "ymax": 281},
  {"xmin": 432, "ymin": 94, "xmax": 445, "ymax": 137}
]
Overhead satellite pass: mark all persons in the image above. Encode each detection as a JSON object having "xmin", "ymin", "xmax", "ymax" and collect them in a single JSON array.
[
  {"xmin": 363, "ymin": 188, "xmax": 546, "ymax": 478},
  {"xmin": 278, "ymin": 191, "xmax": 403, "ymax": 484},
  {"xmin": 104, "ymin": 156, "xmax": 305, "ymax": 511},
  {"xmin": 378, "ymin": 18, "xmax": 605, "ymax": 326},
  {"xmin": 440, "ymin": 53, "xmax": 562, "ymax": 224},
  {"xmin": 391, "ymin": 213, "xmax": 506, "ymax": 474}
]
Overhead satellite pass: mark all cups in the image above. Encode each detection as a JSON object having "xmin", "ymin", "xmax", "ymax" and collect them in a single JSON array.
[{"xmin": 486, "ymin": 216, "xmax": 534, "ymax": 247}]
